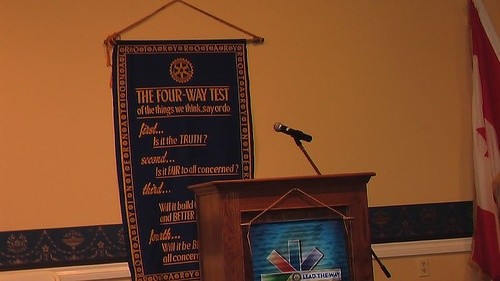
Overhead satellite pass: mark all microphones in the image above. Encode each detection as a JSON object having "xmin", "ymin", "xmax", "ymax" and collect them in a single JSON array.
[{"xmin": 273, "ymin": 121, "xmax": 312, "ymax": 142}]
